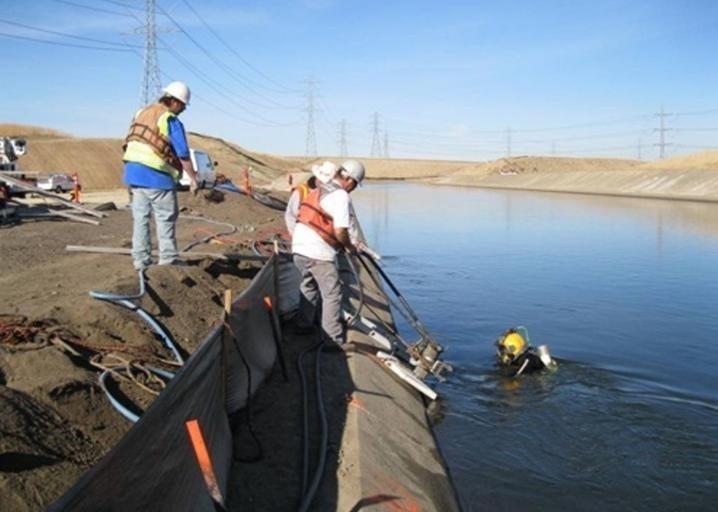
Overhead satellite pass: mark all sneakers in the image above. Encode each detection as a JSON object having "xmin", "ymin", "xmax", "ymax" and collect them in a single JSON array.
[
  {"xmin": 295, "ymin": 327, "xmax": 317, "ymax": 334},
  {"xmin": 322, "ymin": 343, "xmax": 356, "ymax": 352},
  {"xmin": 135, "ymin": 259, "xmax": 189, "ymax": 270}
]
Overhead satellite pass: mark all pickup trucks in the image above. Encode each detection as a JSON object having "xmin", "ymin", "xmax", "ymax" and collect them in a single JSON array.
[{"xmin": 37, "ymin": 173, "xmax": 82, "ymax": 193}]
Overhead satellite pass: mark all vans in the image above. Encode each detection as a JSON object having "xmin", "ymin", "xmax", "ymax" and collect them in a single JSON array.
[{"xmin": 178, "ymin": 149, "xmax": 218, "ymax": 189}]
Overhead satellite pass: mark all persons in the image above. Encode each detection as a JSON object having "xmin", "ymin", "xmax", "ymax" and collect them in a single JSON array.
[
  {"xmin": 283, "ymin": 160, "xmax": 338, "ymax": 237},
  {"xmin": 119, "ymin": 80, "xmax": 201, "ymax": 275},
  {"xmin": 493, "ymin": 328, "xmax": 546, "ymax": 377},
  {"xmin": 290, "ymin": 159, "xmax": 365, "ymax": 353}
]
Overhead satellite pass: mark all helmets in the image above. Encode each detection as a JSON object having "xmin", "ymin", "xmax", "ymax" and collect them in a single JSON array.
[
  {"xmin": 340, "ymin": 160, "xmax": 365, "ymax": 187},
  {"xmin": 161, "ymin": 81, "xmax": 190, "ymax": 106},
  {"xmin": 312, "ymin": 161, "xmax": 338, "ymax": 184},
  {"xmin": 498, "ymin": 333, "xmax": 526, "ymax": 357}
]
